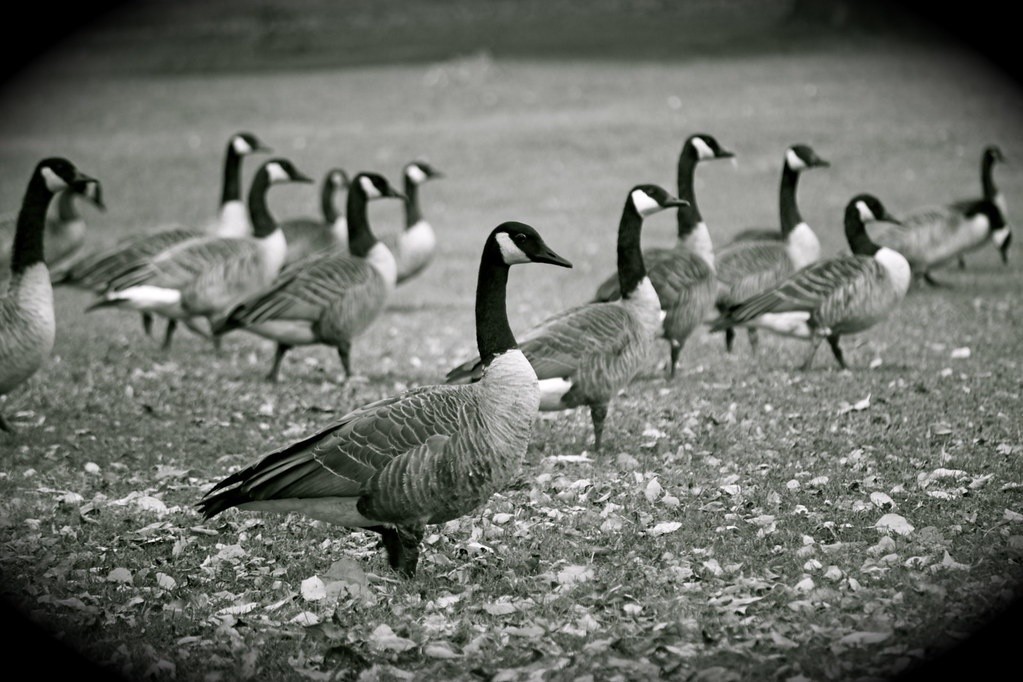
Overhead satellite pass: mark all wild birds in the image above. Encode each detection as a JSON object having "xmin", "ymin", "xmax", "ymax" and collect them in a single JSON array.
[
  {"xmin": 277, "ymin": 167, "xmax": 352, "ymax": 269},
  {"xmin": 378, "ymin": 160, "xmax": 449, "ymax": 286},
  {"xmin": 0, "ymin": 156, "xmax": 101, "ymax": 435},
  {"xmin": 444, "ymin": 132, "xmax": 1012, "ymax": 452},
  {"xmin": 223, "ymin": 171, "xmax": 410, "ymax": 385},
  {"xmin": 82, "ymin": 158, "xmax": 316, "ymax": 356},
  {"xmin": 193, "ymin": 220, "xmax": 573, "ymax": 581},
  {"xmin": 0, "ymin": 179, "xmax": 108, "ymax": 282},
  {"xmin": 52, "ymin": 130, "xmax": 275, "ymax": 342}
]
